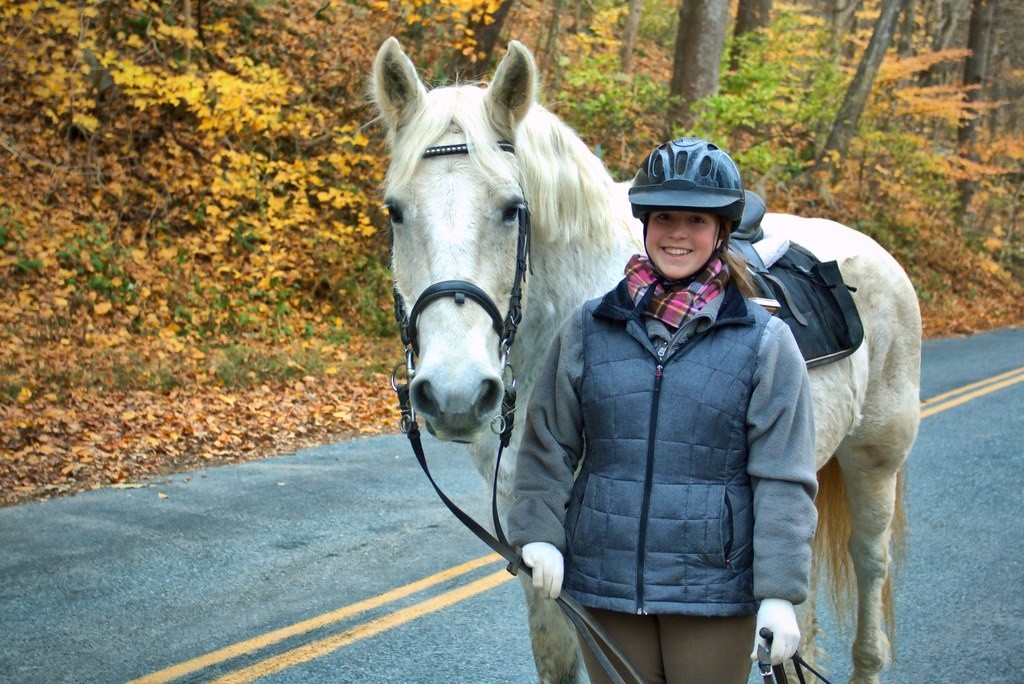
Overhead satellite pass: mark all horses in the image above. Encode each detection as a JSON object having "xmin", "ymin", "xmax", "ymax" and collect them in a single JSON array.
[{"xmin": 370, "ymin": 35, "xmax": 922, "ymax": 684}]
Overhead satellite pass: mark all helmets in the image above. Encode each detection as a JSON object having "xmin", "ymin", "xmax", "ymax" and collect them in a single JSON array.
[{"xmin": 629, "ymin": 138, "xmax": 745, "ymax": 233}]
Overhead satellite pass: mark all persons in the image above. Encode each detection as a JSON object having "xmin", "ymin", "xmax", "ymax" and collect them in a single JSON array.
[{"xmin": 505, "ymin": 136, "xmax": 819, "ymax": 684}]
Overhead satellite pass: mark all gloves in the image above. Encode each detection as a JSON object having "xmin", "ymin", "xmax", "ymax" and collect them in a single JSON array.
[
  {"xmin": 521, "ymin": 541, "xmax": 564, "ymax": 599},
  {"xmin": 750, "ymin": 597, "xmax": 801, "ymax": 665}
]
